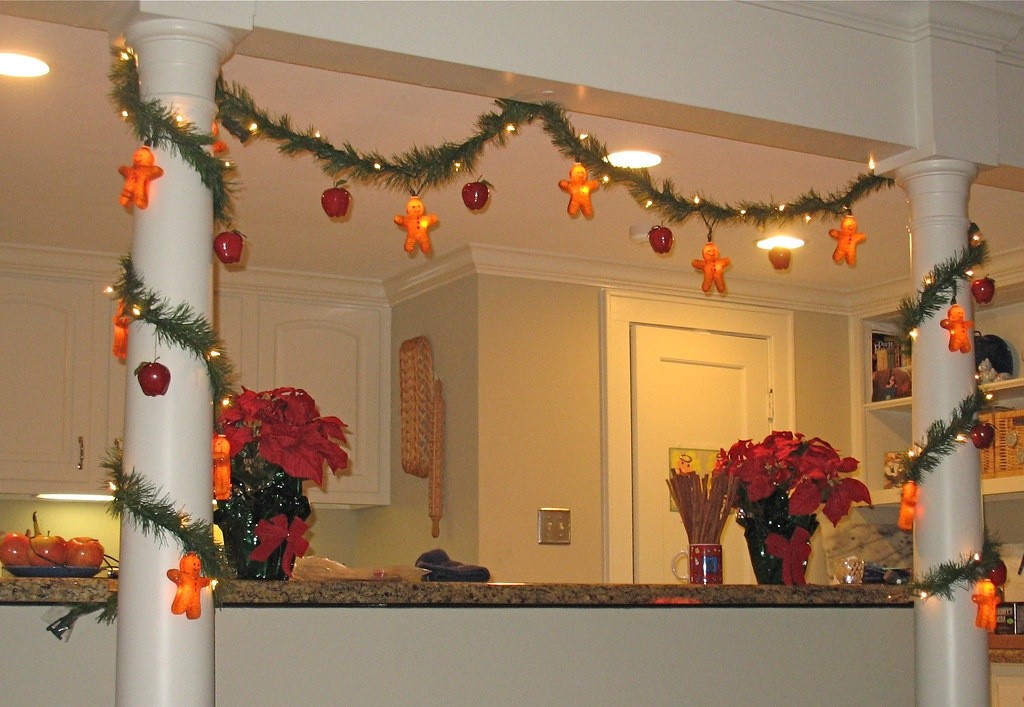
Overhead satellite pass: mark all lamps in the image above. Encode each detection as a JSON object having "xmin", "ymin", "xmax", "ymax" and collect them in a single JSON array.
[
  {"xmin": 29, "ymin": 494, "xmax": 117, "ymax": 501},
  {"xmin": 0, "ymin": 48, "xmax": 53, "ymax": 81},
  {"xmin": 599, "ymin": 147, "xmax": 664, "ymax": 170},
  {"xmin": 756, "ymin": 234, "xmax": 806, "ymax": 250}
]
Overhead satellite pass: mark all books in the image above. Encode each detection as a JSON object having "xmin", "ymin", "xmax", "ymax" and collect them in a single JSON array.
[{"xmin": 870, "ymin": 330, "xmax": 911, "ymax": 371}]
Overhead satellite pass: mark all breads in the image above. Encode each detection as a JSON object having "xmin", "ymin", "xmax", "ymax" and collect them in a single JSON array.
[{"xmin": 292, "ymin": 556, "xmax": 355, "ymax": 579}]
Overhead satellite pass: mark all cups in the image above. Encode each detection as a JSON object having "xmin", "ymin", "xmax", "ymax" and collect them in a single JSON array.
[
  {"xmin": 671, "ymin": 543, "xmax": 723, "ymax": 586},
  {"xmin": 835, "ymin": 560, "xmax": 865, "ymax": 583}
]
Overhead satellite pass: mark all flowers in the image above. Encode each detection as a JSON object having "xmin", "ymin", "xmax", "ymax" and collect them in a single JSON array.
[
  {"xmin": 210, "ymin": 380, "xmax": 366, "ymax": 499},
  {"xmin": 719, "ymin": 430, "xmax": 875, "ymax": 529}
]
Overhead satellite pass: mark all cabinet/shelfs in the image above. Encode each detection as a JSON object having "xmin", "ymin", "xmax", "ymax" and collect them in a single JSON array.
[
  {"xmin": 848, "ymin": 270, "xmax": 1024, "ymax": 508},
  {"xmin": 0, "ymin": 240, "xmax": 392, "ymax": 506}
]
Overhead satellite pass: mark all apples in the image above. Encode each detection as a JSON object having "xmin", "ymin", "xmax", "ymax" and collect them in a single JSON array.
[
  {"xmin": 138, "ymin": 357, "xmax": 171, "ymax": 396},
  {"xmin": 214, "ymin": 231, "xmax": 243, "ymax": 264},
  {"xmin": 0, "ymin": 530, "xmax": 105, "ymax": 569},
  {"xmin": 321, "ymin": 186, "xmax": 349, "ymax": 218},
  {"xmin": 989, "ymin": 560, "xmax": 1006, "ymax": 585},
  {"xmin": 973, "ymin": 274, "xmax": 994, "ymax": 303},
  {"xmin": 970, "ymin": 423, "xmax": 996, "ymax": 450},
  {"xmin": 461, "ymin": 181, "xmax": 489, "ymax": 210},
  {"xmin": 648, "ymin": 225, "xmax": 673, "ymax": 254},
  {"xmin": 768, "ymin": 246, "xmax": 791, "ymax": 270}
]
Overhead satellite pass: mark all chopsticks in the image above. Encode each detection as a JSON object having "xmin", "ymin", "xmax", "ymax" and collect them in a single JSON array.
[{"xmin": 664, "ymin": 466, "xmax": 739, "ymax": 545}]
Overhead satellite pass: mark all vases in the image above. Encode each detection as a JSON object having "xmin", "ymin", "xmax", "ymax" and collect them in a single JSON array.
[
  {"xmin": 744, "ymin": 487, "xmax": 811, "ymax": 586},
  {"xmin": 214, "ymin": 490, "xmax": 312, "ymax": 582}
]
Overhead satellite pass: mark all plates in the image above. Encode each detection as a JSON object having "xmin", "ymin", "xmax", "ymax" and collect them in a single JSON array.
[{"xmin": 3, "ymin": 565, "xmax": 102, "ymax": 577}]
